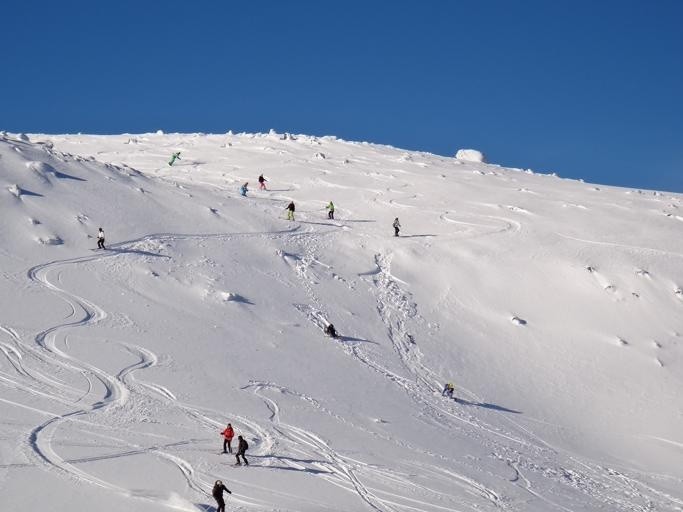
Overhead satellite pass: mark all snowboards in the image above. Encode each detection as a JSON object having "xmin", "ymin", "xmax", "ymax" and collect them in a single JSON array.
[{"xmin": 168, "ymin": 162, "xmax": 173, "ymax": 167}]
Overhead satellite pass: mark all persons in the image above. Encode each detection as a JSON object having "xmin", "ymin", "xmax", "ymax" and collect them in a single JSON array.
[
  {"xmin": 221, "ymin": 423, "xmax": 249, "ymax": 466},
  {"xmin": 322, "ymin": 324, "xmax": 337, "ymax": 338},
  {"xmin": 393, "ymin": 217, "xmax": 400, "ymax": 236},
  {"xmin": 325, "ymin": 200, "xmax": 335, "ymax": 220},
  {"xmin": 257, "ymin": 173, "xmax": 267, "ymax": 191},
  {"xmin": 95, "ymin": 227, "xmax": 105, "ymax": 247},
  {"xmin": 239, "ymin": 181, "xmax": 249, "ymax": 196},
  {"xmin": 286, "ymin": 200, "xmax": 295, "ymax": 221},
  {"xmin": 210, "ymin": 479, "xmax": 233, "ymax": 512},
  {"xmin": 442, "ymin": 382, "xmax": 454, "ymax": 397},
  {"xmin": 168, "ymin": 151, "xmax": 181, "ymax": 166}
]
